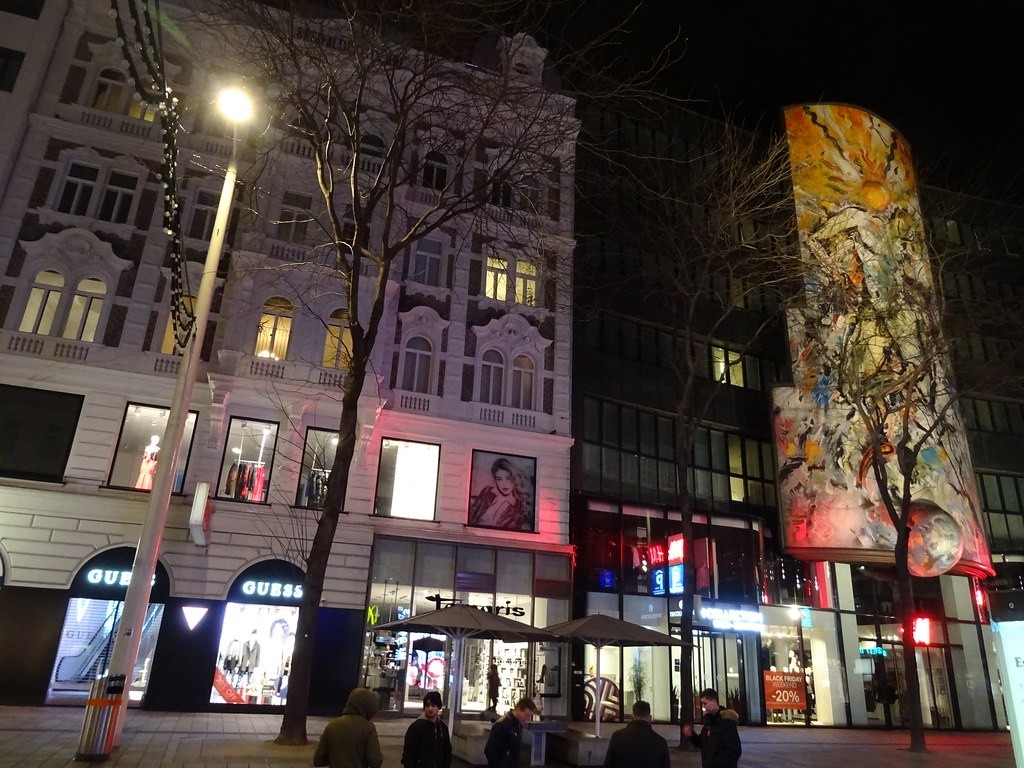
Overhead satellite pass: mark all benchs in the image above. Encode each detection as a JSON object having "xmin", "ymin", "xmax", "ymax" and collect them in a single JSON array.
[
  {"xmin": 451, "ymin": 724, "xmax": 493, "ymax": 765},
  {"xmin": 546, "ymin": 728, "xmax": 610, "ymax": 766}
]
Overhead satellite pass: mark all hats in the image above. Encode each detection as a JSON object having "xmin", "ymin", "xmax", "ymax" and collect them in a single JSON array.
[{"xmin": 423, "ymin": 691, "xmax": 442, "ymax": 707}]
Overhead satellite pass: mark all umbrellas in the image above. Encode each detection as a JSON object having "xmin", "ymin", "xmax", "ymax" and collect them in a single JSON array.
[
  {"xmin": 411, "ymin": 637, "xmax": 446, "ymax": 690},
  {"xmin": 541, "ymin": 614, "xmax": 693, "ymax": 735},
  {"xmin": 372, "ymin": 605, "xmax": 560, "ymax": 739}
]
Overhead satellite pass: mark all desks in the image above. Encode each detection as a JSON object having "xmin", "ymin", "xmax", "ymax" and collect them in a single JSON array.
[{"xmin": 481, "ymin": 710, "xmax": 567, "ymax": 765}]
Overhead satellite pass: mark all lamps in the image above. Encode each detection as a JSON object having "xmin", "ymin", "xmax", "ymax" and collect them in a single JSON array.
[
  {"xmin": 263, "ymin": 425, "xmax": 272, "ymax": 434},
  {"xmin": 241, "ymin": 421, "xmax": 247, "ymax": 429},
  {"xmin": 160, "ymin": 411, "xmax": 167, "ymax": 416},
  {"xmin": 135, "ymin": 406, "xmax": 142, "ymax": 416}
]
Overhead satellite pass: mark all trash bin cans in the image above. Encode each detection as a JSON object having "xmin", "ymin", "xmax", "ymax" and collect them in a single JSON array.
[{"xmin": 71, "ymin": 673, "xmax": 127, "ymax": 761}]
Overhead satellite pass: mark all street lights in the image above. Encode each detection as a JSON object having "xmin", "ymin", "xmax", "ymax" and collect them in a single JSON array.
[{"xmin": 108, "ymin": 84, "xmax": 257, "ymax": 750}]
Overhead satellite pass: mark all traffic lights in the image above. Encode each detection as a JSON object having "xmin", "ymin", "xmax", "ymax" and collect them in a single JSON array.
[
  {"xmin": 976, "ymin": 590, "xmax": 984, "ymax": 606},
  {"xmin": 914, "ymin": 616, "xmax": 931, "ymax": 645}
]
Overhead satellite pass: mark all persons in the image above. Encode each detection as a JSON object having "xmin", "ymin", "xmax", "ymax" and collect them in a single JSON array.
[
  {"xmin": 484, "ymin": 698, "xmax": 536, "ymax": 768},
  {"xmin": 681, "ymin": 688, "xmax": 742, "ymax": 768},
  {"xmin": 315, "ymin": 688, "xmax": 383, "ymax": 768},
  {"xmin": 471, "ymin": 454, "xmax": 536, "ymax": 532},
  {"xmin": 401, "ymin": 691, "xmax": 452, "ymax": 768},
  {"xmin": 277, "ymin": 633, "xmax": 296, "ymax": 697},
  {"xmin": 603, "ymin": 701, "xmax": 671, "ymax": 768},
  {"xmin": 224, "ymin": 629, "xmax": 260, "ymax": 690},
  {"xmin": 488, "ymin": 665, "xmax": 501, "ymax": 712},
  {"xmin": 769, "ymin": 657, "xmax": 815, "ymax": 720},
  {"xmin": 135, "ymin": 435, "xmax": 160, "ymax": 489}
]
